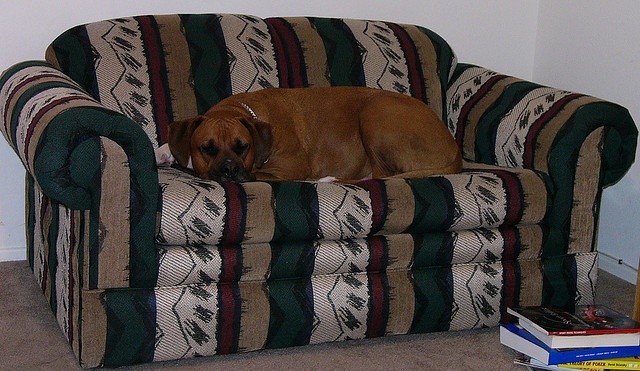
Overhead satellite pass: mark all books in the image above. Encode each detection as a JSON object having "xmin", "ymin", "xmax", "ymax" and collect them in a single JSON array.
[{"xmin": 499, "ymin": 305, "xmax": 640, "ymax": 371}]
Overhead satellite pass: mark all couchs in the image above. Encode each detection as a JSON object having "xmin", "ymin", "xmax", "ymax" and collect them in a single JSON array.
[{"xmin": 1, "ymin": 13, "xmax": 636, "ymax": 371}]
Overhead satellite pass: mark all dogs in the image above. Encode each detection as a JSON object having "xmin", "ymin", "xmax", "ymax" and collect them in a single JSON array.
[{"xmin": 166, "ymin": 85, "xmax": 463, "ymax": 184}]
